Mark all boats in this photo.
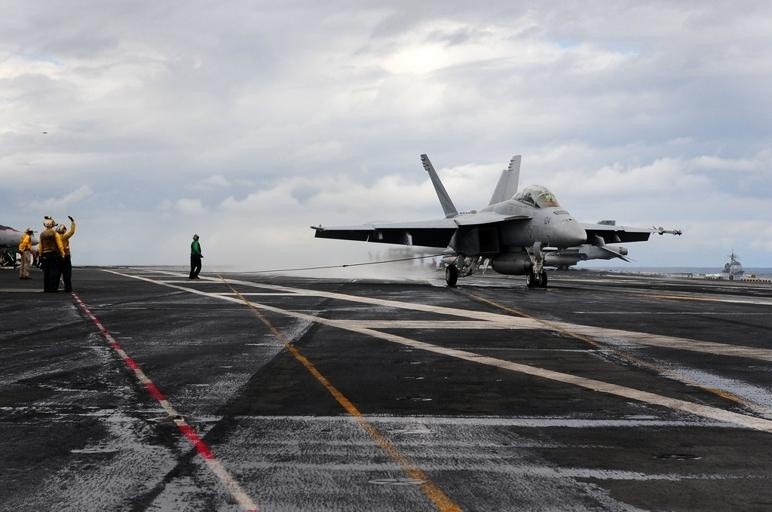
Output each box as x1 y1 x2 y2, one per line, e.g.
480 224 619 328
725 251 744 274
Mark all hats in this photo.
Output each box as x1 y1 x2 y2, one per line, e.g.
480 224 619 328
25 227 34 233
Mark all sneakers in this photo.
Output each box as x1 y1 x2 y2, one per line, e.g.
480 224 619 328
190 272 200 280
18 274 32 279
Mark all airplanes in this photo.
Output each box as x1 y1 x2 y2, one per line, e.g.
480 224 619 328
0 225 42 265
310 154 683 286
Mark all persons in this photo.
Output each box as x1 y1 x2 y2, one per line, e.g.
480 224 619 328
188 234 203 280
18 215 76 294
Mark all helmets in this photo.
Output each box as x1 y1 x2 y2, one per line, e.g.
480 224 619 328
43 218 67 233
192 234 200 240
